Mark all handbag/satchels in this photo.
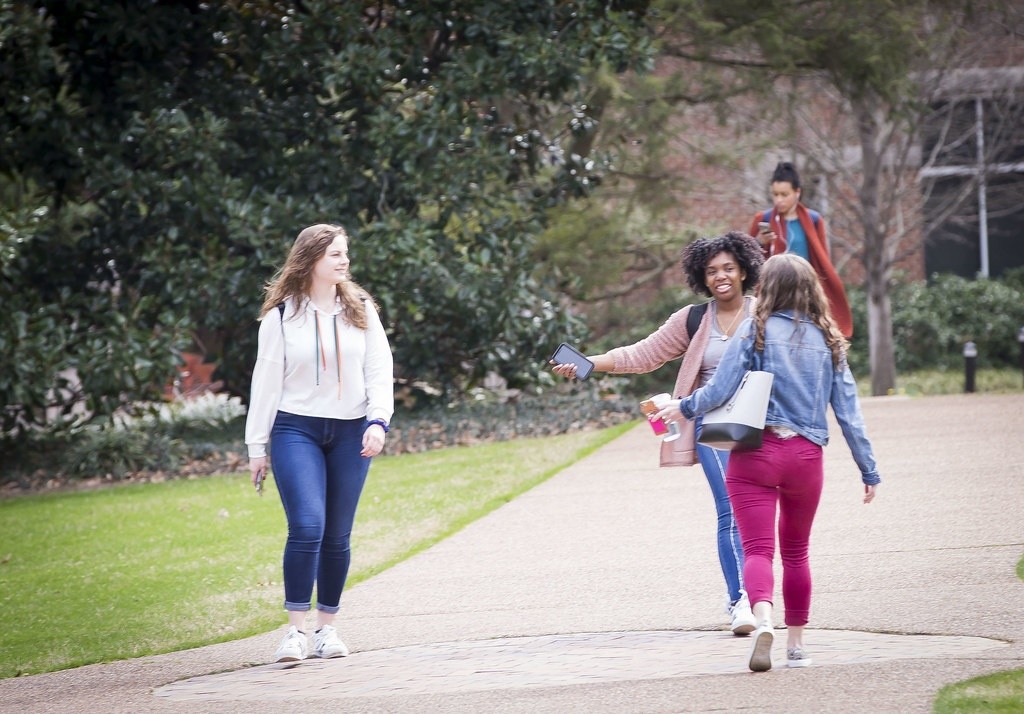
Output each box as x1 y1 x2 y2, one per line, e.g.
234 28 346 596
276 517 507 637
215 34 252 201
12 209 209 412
697 369 775 453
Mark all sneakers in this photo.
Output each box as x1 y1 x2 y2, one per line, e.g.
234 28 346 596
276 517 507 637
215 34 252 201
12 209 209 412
745 620 774 673
272 625 309 663
727 590 757 636
306 624 349 660
784 645 810 668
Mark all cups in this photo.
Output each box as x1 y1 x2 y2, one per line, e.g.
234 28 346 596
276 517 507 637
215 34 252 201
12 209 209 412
647 392 681 443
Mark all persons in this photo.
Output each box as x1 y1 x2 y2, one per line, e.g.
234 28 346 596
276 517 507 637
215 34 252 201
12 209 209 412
651 254 881 672
549 232 761 636
242 224 394 664
747 162 855 341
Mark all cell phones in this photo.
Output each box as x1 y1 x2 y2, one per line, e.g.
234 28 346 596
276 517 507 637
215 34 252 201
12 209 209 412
640 400 668 438
757 222 770 233
257 472 263 497
551 342 594 382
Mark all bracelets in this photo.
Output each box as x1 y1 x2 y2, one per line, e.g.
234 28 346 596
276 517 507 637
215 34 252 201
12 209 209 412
366 420 390 433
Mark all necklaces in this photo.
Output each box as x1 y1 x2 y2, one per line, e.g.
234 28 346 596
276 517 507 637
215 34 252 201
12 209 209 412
715 295 746 342
